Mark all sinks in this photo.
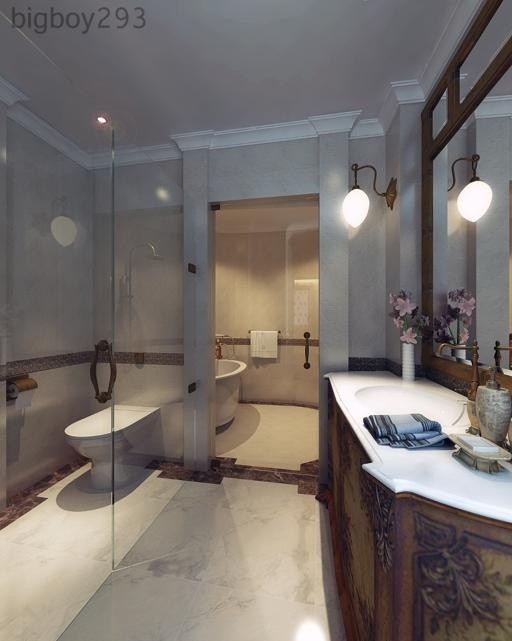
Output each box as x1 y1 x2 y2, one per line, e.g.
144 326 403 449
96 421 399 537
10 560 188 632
354 384 468 438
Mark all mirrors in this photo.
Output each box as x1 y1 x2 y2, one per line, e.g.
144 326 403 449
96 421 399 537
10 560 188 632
420 1 512 376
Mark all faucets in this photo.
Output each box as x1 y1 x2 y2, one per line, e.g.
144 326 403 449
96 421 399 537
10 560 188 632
492 340 511 373
437 338 480 402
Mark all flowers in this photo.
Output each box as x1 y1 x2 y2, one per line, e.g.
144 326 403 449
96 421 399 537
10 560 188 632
433 286 479 348
388 288 431 344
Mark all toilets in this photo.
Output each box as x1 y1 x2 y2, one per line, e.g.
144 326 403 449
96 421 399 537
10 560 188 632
63 403 161 491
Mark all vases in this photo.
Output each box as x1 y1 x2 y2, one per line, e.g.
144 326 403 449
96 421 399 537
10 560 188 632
449 340 469 359
399 335 415 380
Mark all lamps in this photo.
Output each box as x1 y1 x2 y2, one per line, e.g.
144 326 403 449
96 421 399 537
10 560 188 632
342 163 396 228
447 153 497 221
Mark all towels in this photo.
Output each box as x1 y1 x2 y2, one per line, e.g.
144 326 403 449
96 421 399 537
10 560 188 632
249 330 278 358
363 413 457 450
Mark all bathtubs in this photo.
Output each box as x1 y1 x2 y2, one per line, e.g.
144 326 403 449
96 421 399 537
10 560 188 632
215 357 248 434
